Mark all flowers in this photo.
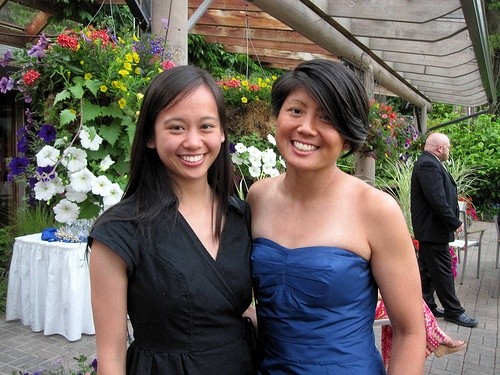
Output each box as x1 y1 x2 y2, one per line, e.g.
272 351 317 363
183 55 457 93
0 18 419 227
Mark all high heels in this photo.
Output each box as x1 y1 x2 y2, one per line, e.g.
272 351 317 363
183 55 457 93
434 339 467 358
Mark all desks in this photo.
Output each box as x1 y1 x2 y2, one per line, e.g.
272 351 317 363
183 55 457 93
6 232 136 341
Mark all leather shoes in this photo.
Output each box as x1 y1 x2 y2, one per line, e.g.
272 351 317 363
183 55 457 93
433 304 479 327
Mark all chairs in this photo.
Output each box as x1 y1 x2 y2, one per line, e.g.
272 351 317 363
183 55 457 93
449 210 487 285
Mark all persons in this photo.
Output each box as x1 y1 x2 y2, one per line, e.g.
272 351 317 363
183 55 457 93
410 132 479 328
371 285 467 375
87 66 269 375
245 59 427 375
